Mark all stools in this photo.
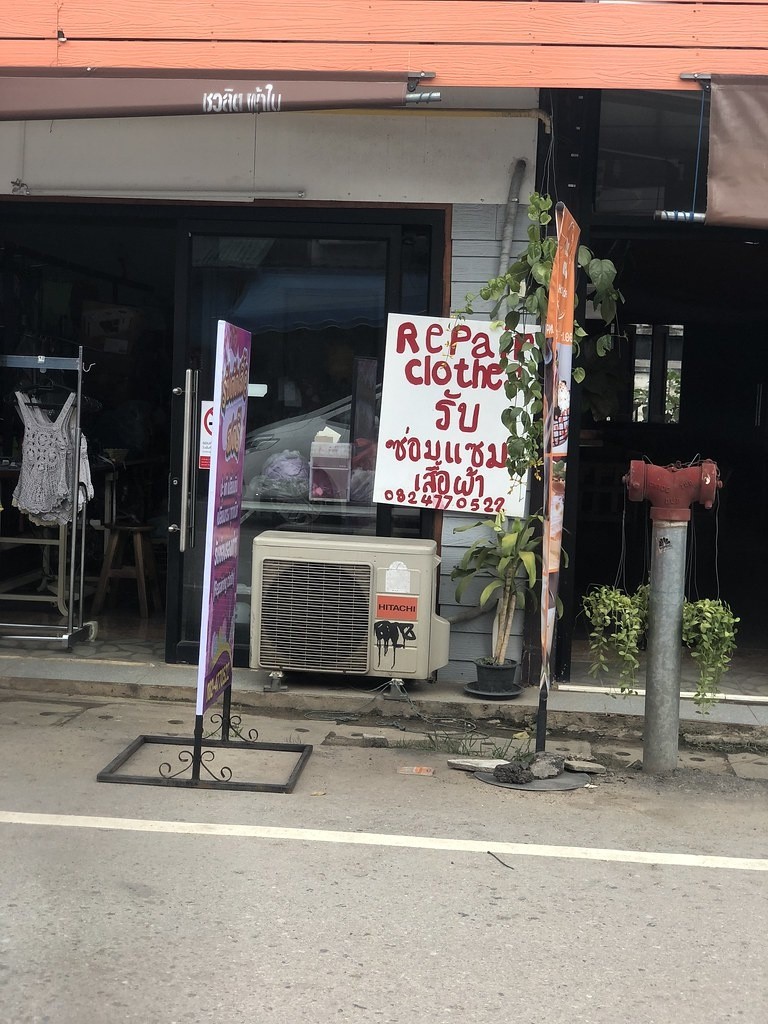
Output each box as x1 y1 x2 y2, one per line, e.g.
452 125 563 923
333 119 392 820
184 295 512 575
90 521 164 623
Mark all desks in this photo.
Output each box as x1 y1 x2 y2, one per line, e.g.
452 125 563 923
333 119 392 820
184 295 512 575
0 452 160 600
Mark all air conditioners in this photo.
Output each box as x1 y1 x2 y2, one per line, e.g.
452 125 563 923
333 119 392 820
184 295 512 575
247 528 452 681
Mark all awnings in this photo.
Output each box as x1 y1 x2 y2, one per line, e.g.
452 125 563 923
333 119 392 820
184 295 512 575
653 71 767 228
1 64 441 120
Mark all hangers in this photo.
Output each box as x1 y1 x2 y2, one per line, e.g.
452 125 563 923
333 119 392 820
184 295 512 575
4 364 104 411
